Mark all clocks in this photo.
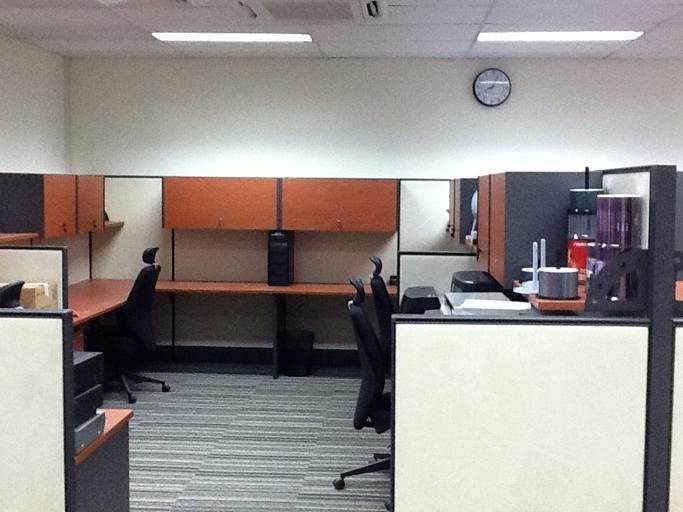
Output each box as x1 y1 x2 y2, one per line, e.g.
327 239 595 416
473 68 512 107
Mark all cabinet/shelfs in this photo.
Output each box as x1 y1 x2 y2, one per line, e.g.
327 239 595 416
161 175 279 233
477 171 603 290
0 172 105 239
278 176 400 234
447 179 478 244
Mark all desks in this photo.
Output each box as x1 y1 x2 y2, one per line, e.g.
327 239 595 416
74 408 134 512
68 278 397 379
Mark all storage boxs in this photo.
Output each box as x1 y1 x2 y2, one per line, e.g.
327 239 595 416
19 280 58 311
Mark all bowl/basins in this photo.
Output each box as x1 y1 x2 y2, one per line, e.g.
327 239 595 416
537 267 578 298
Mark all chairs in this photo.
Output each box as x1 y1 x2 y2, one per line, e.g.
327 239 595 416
369 255 398 379
89 246 171 403
333 277 393 510
